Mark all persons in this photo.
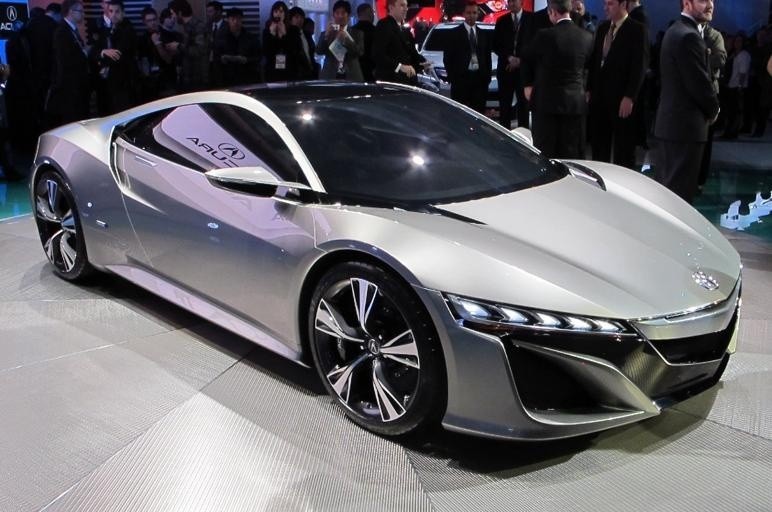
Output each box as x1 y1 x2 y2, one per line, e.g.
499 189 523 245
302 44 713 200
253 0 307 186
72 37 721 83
0 1 771 206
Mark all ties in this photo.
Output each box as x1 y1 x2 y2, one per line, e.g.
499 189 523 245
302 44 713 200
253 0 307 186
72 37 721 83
514 14 518 31
470 28 476 54
601 24 616 67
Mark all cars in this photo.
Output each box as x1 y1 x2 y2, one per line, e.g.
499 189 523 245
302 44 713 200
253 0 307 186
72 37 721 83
30 79 744 450
417 19 517 120
